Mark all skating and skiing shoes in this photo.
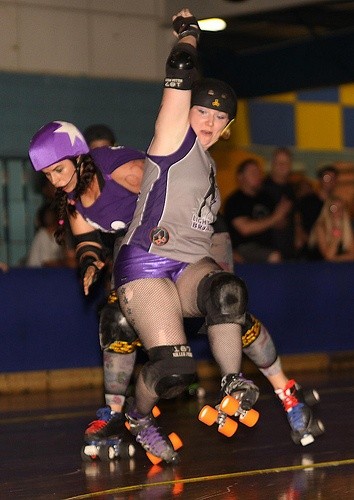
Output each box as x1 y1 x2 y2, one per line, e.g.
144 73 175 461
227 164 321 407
83 407 138 460
274 379 324 446
199 373 260 437
125 406 183 465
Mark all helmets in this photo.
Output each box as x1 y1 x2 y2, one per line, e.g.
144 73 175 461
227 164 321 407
191 78 237 120
29 121 90 172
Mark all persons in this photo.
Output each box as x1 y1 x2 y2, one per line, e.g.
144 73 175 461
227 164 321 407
84 125 116 150
27 205 65 269
26 120 320 461
112 10 260 468
217 146 354 263
